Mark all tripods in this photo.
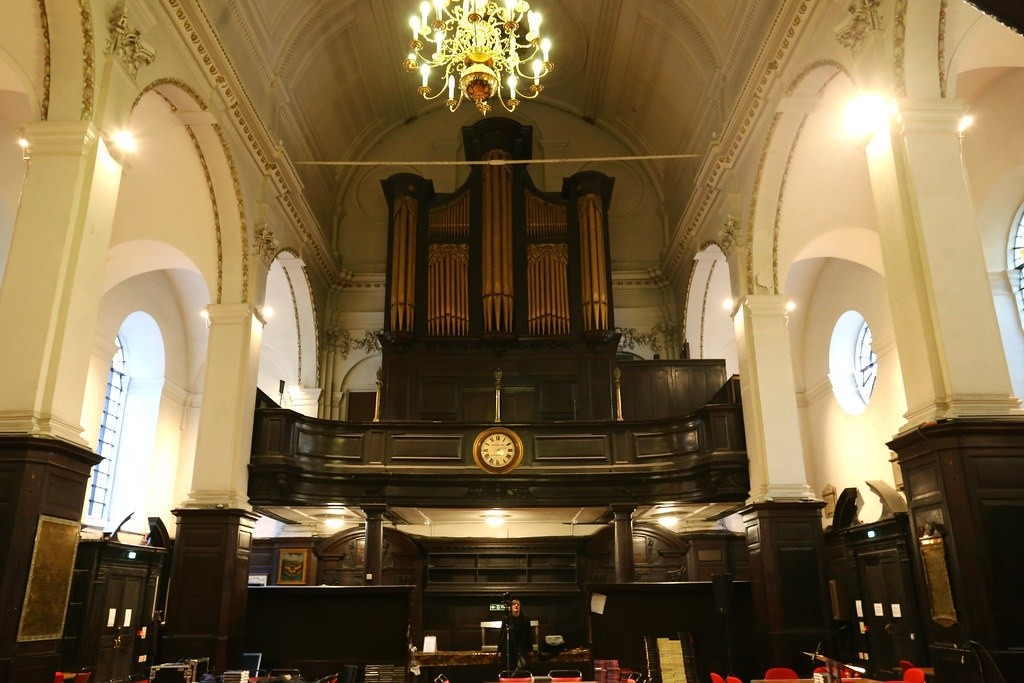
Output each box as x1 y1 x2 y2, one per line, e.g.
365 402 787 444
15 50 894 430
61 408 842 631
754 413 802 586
484 618 525 683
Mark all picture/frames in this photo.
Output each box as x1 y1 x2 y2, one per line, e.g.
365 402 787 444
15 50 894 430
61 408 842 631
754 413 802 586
275 549 308 584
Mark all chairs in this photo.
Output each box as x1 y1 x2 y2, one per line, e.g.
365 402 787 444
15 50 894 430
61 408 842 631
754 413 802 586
55 667 339 683
433 670 643 683
710 661 925 683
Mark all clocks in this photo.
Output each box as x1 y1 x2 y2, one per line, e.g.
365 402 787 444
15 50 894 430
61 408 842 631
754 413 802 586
473 427 524 474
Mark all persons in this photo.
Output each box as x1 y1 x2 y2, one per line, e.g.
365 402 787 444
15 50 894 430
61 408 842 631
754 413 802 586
498 598 536 674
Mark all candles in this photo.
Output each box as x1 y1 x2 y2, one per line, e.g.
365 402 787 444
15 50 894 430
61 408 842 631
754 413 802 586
409 0 553 102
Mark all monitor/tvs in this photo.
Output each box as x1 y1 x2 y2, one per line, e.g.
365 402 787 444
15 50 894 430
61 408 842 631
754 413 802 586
101 512 134 534
866 480 907 514
148 517 170 549
832 487 865 527
